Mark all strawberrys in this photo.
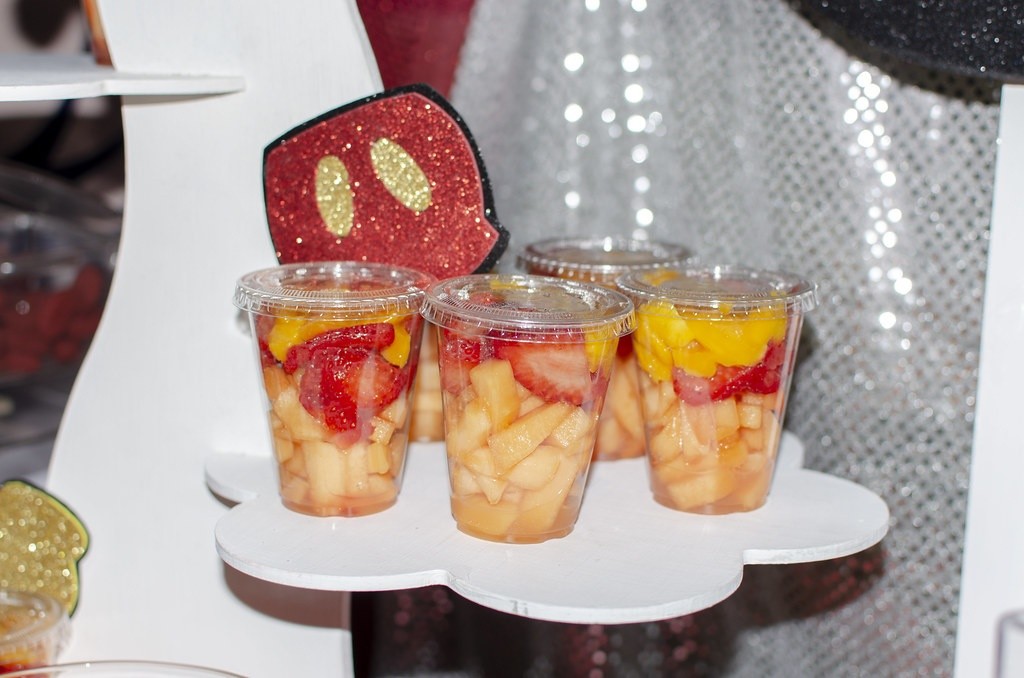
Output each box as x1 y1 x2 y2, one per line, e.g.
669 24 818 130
255 281 792 433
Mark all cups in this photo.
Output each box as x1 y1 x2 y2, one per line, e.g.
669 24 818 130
0 589 65 678
234 235 819 545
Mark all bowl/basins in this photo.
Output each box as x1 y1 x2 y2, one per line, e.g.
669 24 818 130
0 661 246 678
